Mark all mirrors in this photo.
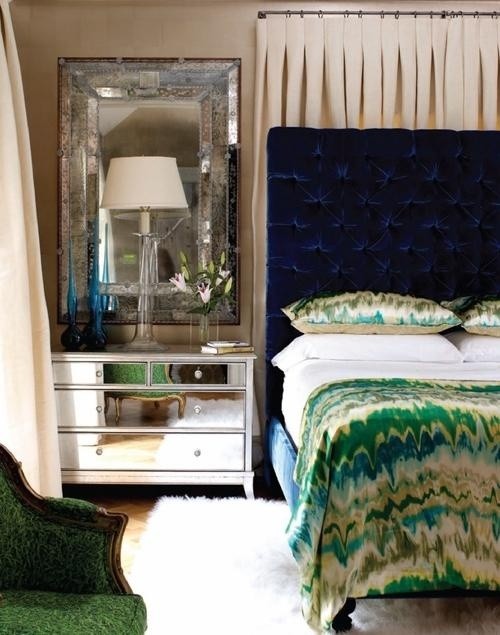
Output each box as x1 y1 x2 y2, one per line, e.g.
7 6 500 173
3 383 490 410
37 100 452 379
56 58 239 326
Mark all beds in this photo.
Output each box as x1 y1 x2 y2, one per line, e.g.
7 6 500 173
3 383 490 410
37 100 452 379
267 363 498 632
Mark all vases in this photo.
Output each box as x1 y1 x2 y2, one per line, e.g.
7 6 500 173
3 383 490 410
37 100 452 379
199 311 218 344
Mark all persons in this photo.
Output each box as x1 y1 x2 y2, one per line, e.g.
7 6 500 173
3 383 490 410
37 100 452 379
156 247 179 283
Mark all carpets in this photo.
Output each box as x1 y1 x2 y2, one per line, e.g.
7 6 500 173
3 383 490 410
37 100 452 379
141 489 498 632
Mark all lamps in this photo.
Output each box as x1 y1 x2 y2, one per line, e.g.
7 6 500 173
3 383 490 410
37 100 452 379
99 156 190 353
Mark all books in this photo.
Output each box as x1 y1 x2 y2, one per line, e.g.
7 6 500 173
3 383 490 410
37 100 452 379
208 340 250 348
199 344 254 355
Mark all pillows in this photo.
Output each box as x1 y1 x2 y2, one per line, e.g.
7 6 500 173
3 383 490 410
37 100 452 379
274 291 499 367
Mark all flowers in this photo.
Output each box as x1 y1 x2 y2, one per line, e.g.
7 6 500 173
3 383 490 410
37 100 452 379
174 249 238 314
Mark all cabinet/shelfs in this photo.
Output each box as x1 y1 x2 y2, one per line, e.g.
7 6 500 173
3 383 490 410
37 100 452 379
53 345 258 500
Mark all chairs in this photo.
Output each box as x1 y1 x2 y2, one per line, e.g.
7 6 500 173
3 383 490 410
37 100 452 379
0 446 146 632
